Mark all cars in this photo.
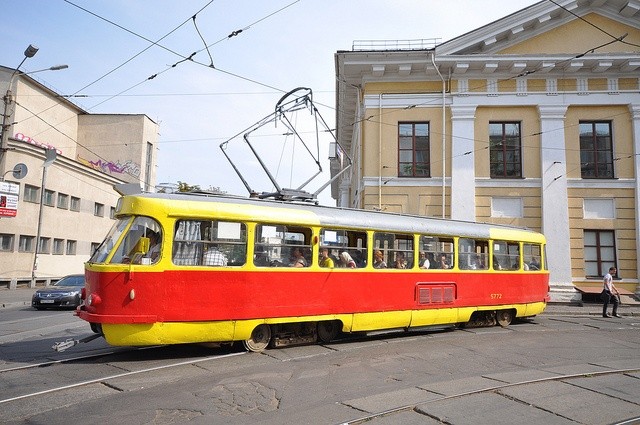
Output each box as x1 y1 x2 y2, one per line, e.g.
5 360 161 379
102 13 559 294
31 274 85 310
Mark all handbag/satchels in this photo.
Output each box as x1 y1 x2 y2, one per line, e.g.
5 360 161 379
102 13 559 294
600 284 611 303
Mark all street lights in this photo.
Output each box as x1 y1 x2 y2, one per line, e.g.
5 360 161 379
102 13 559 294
1 64 69 157
0 43 39 156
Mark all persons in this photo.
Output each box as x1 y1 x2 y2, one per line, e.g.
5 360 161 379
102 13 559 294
427 252 435 269
475 256 485 269
602 267 622 318
335 247 365 268
435 253 449 268
529 256 540 270
373 250 387 268
203 243 228 266
515 257 529 270
140 230 162 264
392 251 409 269
292 246 308 267
254 246 266 266
418 251 431 269
318 249 335 268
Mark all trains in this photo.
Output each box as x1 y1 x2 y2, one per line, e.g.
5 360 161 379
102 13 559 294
75 183 550 354
